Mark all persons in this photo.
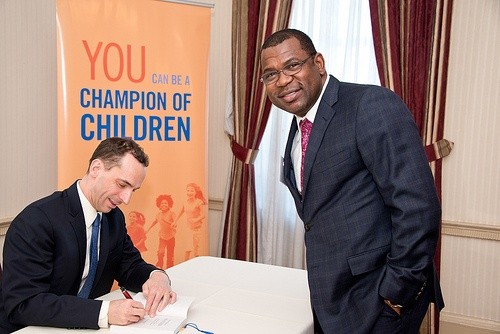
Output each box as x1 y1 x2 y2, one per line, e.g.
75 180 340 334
127 183 207 268
0 137 176 334
260 29 442 334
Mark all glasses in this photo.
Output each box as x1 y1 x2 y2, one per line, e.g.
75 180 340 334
260 53 316 86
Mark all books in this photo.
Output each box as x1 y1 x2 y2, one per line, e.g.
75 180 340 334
109 290 195 334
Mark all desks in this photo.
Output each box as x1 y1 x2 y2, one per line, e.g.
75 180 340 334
8 256 314 334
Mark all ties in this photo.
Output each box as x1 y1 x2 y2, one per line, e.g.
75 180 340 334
77 213 100 299
299 118 313 200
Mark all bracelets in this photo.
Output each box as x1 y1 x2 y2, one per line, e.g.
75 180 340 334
385 300 402 307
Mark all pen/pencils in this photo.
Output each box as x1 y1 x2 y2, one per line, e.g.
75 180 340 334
120 286 132 299
